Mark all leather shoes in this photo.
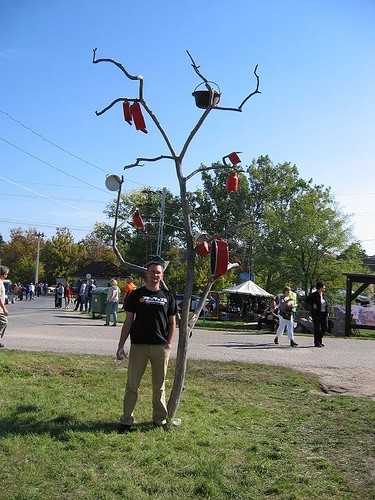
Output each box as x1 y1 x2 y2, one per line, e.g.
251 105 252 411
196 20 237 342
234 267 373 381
315 341 324 346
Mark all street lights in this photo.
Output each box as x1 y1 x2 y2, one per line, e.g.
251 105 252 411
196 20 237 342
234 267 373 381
35 231 45 284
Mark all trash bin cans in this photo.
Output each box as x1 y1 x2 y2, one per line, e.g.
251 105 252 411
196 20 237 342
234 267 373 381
90 287 120 319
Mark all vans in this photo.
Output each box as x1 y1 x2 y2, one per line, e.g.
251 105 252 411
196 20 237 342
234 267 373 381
3 280 16 304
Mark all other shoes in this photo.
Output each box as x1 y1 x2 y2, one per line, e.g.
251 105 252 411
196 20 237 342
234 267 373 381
274 337 279 344
104 323 109 325
290 339 299 347
118 424 133 433
112 323 117 326
157 424 168 430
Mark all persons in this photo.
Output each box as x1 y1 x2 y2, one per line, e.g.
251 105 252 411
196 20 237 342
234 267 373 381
104 279 120 326
35 281 48 297
0 265 10 347
304 281 329 347
116 254 178 433
274 286 298 347
54 282 73 309
121 276 136 303
258 283 297 332
26 283 35 301
212 303 240 316
9 282 24 304
3 282 8 305
74 279 96 313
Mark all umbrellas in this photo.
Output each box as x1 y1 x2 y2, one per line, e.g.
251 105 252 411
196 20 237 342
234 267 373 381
220 280 275 310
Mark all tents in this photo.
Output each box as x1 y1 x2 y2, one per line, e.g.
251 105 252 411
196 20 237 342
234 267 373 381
340 290 371 305
